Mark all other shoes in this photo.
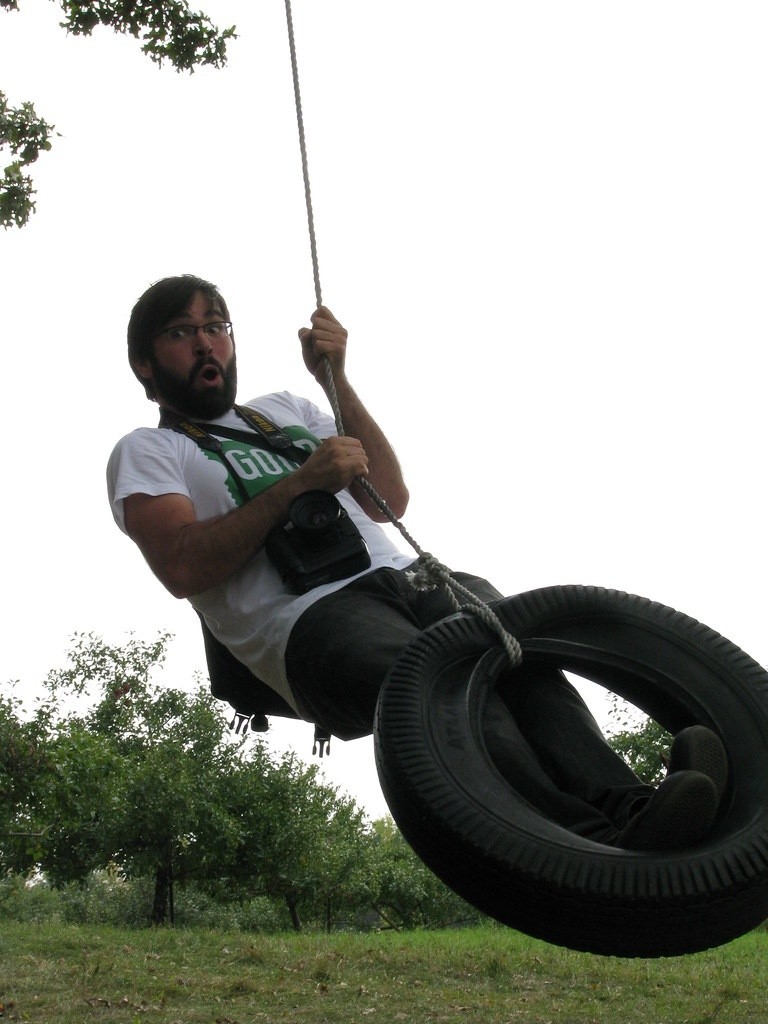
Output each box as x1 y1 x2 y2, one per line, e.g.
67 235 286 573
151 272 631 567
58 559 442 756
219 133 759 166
615 770 719 852
665 725 728 798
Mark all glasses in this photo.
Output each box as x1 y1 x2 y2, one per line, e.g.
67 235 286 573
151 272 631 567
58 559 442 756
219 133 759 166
149 320 232 343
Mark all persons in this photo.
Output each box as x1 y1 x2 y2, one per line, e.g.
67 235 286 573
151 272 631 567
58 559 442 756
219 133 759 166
107 274 728 852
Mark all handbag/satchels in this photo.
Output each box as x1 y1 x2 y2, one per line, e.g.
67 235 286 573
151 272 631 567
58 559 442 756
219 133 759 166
192 606 331 759
